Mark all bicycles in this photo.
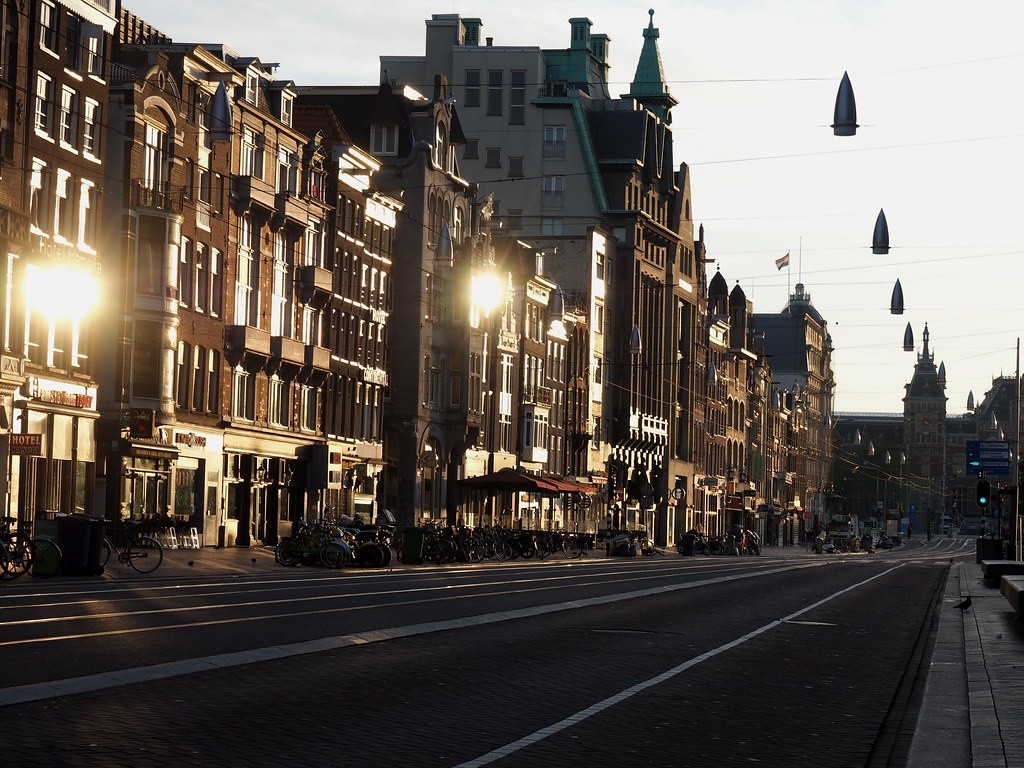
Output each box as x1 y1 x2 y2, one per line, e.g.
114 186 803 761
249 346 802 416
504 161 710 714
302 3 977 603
277 505 397 569
78 512 164 574
402 520 587 564
673 527 729 555
0 514 64 579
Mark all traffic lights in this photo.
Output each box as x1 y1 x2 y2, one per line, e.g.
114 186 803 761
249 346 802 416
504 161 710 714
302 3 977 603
976 480 991 509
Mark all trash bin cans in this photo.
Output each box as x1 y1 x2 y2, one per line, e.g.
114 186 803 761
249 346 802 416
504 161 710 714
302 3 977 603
35 510 106 577
975 536 1003 564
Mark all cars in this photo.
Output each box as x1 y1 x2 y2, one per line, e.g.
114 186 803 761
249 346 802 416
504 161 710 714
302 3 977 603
983 533 1000 540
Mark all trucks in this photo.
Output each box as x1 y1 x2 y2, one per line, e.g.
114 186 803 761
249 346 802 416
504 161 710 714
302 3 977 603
938 516 961 534
828 514 869 540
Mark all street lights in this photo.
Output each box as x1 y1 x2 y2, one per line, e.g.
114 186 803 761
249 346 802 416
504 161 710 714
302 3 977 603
997 488 1008 540
550 320 573 530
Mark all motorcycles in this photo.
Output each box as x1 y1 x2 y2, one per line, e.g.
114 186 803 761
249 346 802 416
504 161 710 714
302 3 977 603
876 535 894 548
